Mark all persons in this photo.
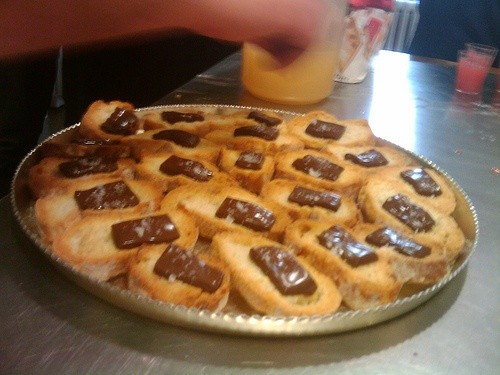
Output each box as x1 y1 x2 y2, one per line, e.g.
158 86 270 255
1 1 342 215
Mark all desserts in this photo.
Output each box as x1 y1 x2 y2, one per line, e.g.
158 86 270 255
31 98 467 320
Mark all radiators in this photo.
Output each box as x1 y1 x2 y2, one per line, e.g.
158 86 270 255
380 1 420 53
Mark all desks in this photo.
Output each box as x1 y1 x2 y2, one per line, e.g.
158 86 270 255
1 44 499 375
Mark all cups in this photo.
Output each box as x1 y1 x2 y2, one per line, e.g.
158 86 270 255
240 0 347 106
456 49 492 95
465 42 498 70
